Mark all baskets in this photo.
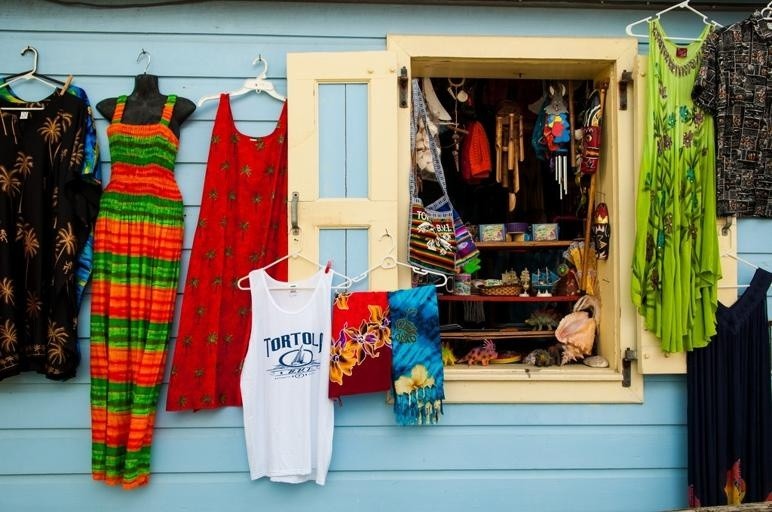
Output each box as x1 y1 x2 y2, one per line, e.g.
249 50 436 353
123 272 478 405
477 283 523 296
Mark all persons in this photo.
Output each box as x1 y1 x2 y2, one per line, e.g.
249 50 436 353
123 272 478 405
95 74 197 488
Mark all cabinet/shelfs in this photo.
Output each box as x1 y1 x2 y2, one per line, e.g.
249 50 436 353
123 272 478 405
412 225 612 372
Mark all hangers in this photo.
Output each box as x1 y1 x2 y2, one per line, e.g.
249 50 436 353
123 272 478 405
759 1 772 23
0 45 80 115
712 228 772 289
334 231 450 292
192 51 289 119
238 228 353 293
627 1 727 42
